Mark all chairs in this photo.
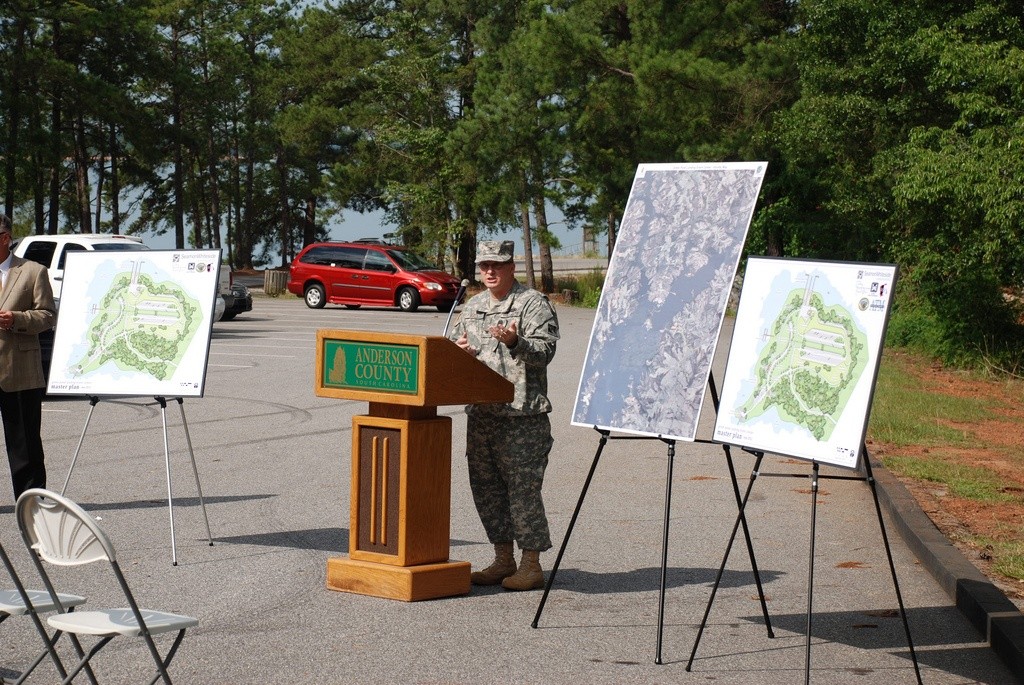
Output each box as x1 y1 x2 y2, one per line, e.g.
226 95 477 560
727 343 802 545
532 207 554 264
0 541 87 685
15 488 199 685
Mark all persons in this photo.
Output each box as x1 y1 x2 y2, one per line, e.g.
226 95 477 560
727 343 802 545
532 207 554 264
0 215 58 505
449 239 562 588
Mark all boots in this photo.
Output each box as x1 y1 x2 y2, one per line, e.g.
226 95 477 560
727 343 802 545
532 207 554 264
470 540 544 589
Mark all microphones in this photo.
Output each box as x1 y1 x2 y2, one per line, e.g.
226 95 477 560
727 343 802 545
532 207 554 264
455 279 470 302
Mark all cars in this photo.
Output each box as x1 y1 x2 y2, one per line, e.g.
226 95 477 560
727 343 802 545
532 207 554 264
220 281 253 320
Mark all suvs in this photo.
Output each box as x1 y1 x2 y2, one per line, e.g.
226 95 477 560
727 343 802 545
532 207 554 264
14 233 225 324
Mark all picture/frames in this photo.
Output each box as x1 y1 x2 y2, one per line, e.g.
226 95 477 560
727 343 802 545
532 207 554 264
46 248 223 399
712 255 899 471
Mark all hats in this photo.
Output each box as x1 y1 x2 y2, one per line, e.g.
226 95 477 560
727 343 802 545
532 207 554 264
474 239 515 263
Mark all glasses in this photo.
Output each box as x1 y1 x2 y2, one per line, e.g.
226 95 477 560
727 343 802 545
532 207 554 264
478 262 503 269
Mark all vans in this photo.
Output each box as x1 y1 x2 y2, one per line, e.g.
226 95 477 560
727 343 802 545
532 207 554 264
288 240 467 313
